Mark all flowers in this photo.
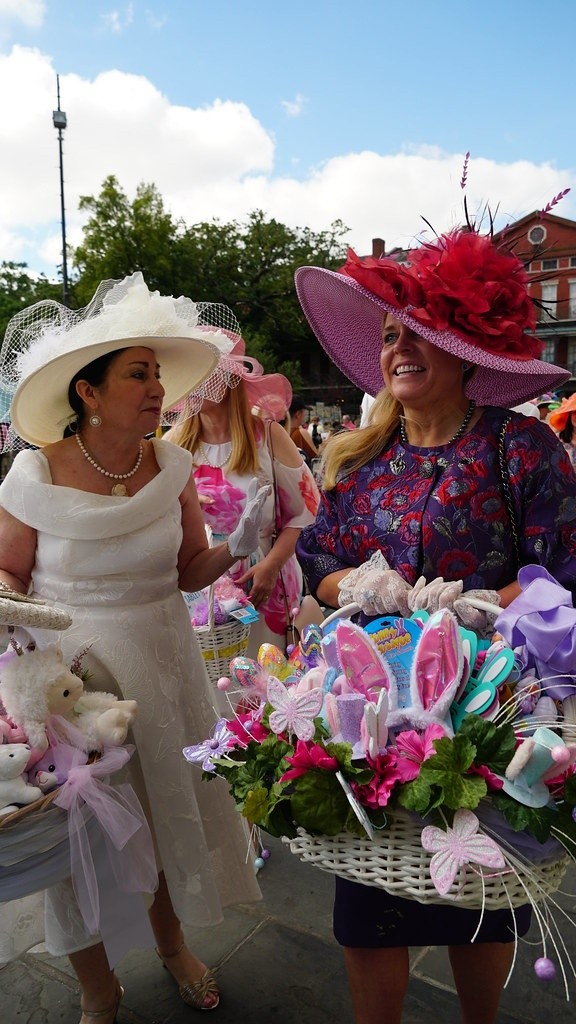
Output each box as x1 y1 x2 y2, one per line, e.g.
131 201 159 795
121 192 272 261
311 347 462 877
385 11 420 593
343 226 555 356
185 668 575 837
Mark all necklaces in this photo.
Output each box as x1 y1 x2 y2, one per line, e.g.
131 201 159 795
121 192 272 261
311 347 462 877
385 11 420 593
199 439 234 470
400 396 476 449
75 430 144 479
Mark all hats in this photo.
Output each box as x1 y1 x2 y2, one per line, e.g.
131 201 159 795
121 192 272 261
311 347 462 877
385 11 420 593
160 327 291 426
294 266 571 412
551 395 576 430
10 313 221 449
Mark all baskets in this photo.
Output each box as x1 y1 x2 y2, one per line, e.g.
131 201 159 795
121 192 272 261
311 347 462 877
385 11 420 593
0 638 106 903
188 579 246 688
287 597 569 908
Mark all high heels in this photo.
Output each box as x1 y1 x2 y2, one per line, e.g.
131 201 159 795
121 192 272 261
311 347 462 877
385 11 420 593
78 972 124 1024
156 938 219 1011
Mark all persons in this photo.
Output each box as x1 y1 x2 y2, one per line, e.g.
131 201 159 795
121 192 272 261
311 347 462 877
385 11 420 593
0 343 274 1024
157 300 324 861
0 392 576 600
293 315 576 1024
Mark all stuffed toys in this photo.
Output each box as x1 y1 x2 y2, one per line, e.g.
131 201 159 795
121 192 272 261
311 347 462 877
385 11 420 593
0 643 137 816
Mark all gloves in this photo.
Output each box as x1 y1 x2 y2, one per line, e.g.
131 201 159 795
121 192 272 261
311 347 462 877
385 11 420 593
227 477 270 558
